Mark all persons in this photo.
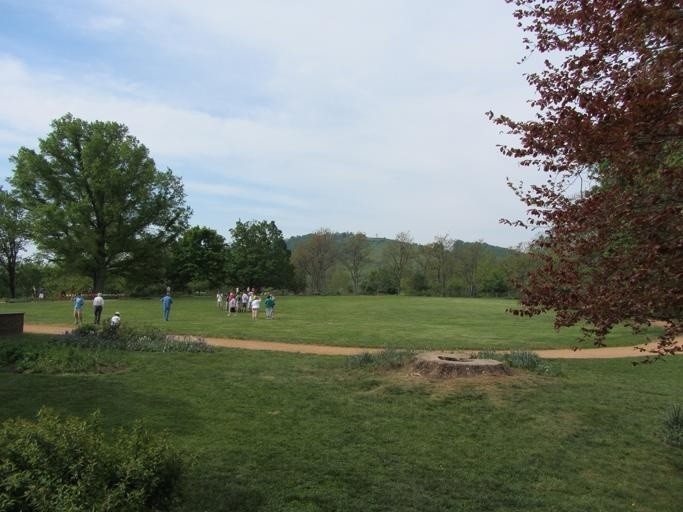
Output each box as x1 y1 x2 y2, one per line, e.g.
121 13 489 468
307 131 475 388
111 312 121 327
216 287 275 320
72 293 84 324
93 293 104 325
160 292 173 321
